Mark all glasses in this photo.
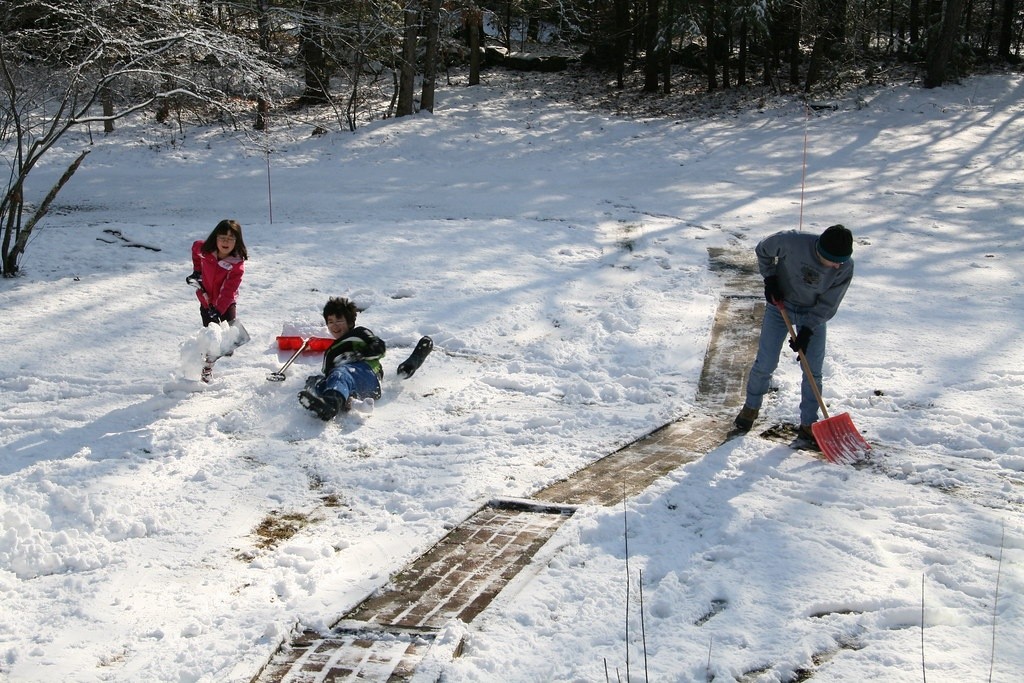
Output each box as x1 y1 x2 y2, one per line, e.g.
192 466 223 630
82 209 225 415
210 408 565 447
217 236 236 244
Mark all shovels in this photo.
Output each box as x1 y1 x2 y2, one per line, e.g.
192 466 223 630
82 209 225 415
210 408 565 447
185 271 253 367
764 293 874 467
267 330 338 387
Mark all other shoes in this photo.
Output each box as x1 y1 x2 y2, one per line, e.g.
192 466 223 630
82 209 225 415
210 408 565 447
798 423 817 445
201 367 212 383
298 390 336 422
734 404 759 432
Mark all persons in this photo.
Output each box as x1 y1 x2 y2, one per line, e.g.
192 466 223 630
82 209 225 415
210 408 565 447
186 220 248 382
298 296 386 421
734 224 854 439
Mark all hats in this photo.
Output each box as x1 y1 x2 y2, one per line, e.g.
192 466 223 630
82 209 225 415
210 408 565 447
817 224 853 263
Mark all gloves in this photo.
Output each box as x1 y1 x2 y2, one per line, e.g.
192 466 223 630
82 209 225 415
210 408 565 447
788 324 813 362
207 308 221 322
763 276 782 304
186 272 198 284
